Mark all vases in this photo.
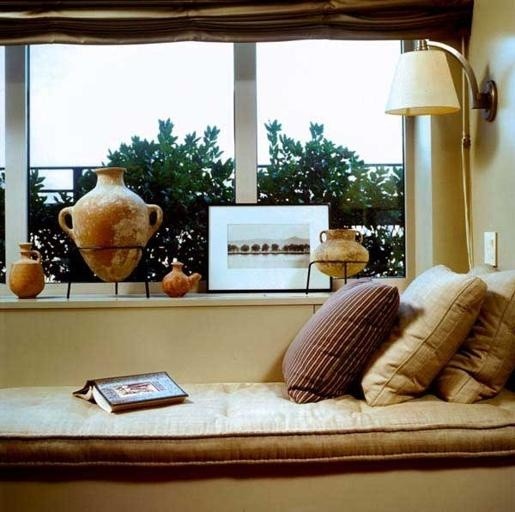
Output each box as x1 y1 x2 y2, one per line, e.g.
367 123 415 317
161 261 201 298
313 227 370 278
59 165 166 285
8 243 46 300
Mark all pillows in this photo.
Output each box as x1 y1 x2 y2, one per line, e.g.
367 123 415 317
367 263 479 407
446 271 515 404
277 277 399 404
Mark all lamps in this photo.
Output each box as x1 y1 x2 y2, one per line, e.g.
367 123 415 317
385 36 499 127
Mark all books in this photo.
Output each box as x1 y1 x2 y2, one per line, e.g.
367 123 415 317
72 367 190 415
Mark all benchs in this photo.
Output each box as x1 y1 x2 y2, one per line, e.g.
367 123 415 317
0 381 515 511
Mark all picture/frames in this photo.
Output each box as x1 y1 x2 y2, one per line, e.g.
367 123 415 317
206 201 332 290
